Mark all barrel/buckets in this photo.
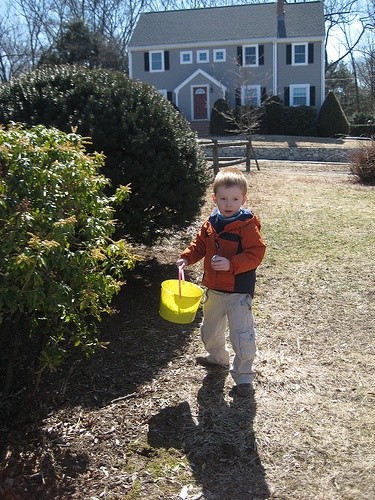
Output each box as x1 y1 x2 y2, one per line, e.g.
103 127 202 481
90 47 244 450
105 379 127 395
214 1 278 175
160 265 204 323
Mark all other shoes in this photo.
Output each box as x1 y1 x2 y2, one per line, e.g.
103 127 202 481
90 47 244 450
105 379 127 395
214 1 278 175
236 383 254 398
194 354 221 366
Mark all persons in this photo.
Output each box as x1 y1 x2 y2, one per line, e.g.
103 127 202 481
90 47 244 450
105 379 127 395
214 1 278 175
176 166 266 396
